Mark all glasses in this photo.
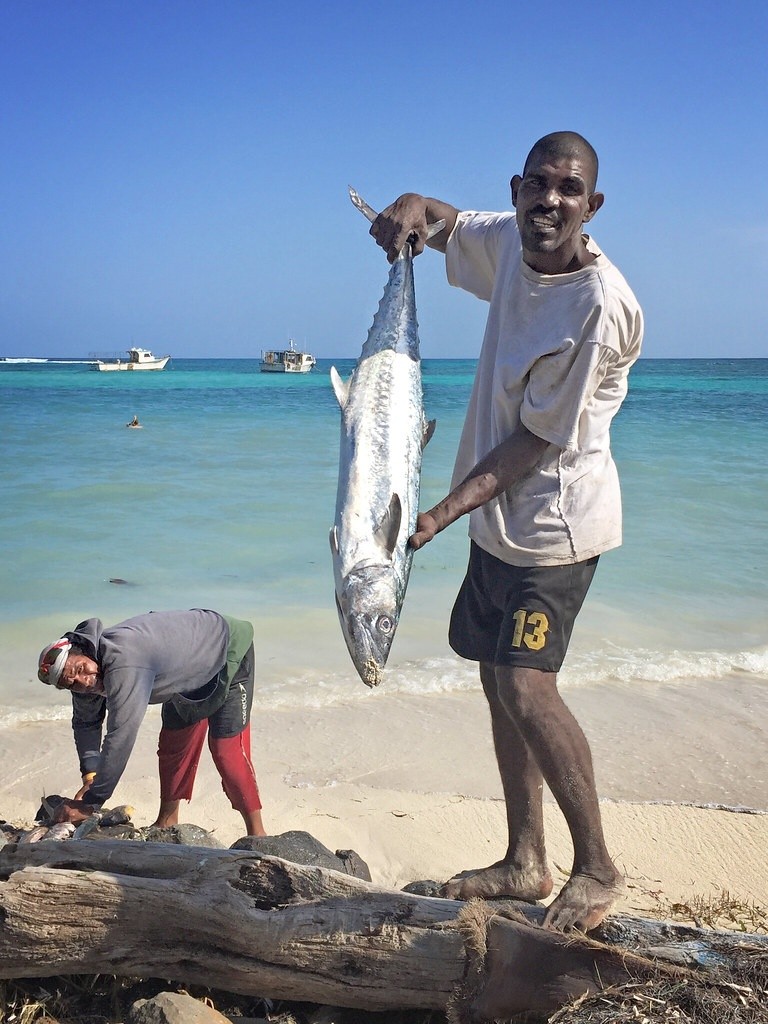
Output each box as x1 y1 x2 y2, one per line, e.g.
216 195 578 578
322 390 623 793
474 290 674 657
38 641 69 685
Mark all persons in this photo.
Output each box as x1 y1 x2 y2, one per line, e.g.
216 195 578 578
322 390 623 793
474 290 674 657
38 608 268 835
368 132 646 933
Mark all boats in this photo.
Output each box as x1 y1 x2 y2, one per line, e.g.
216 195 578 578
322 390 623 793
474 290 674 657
258 340 317 374
87 348 171 371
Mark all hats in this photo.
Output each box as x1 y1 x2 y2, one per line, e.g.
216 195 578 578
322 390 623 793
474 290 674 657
38 638 73 690
